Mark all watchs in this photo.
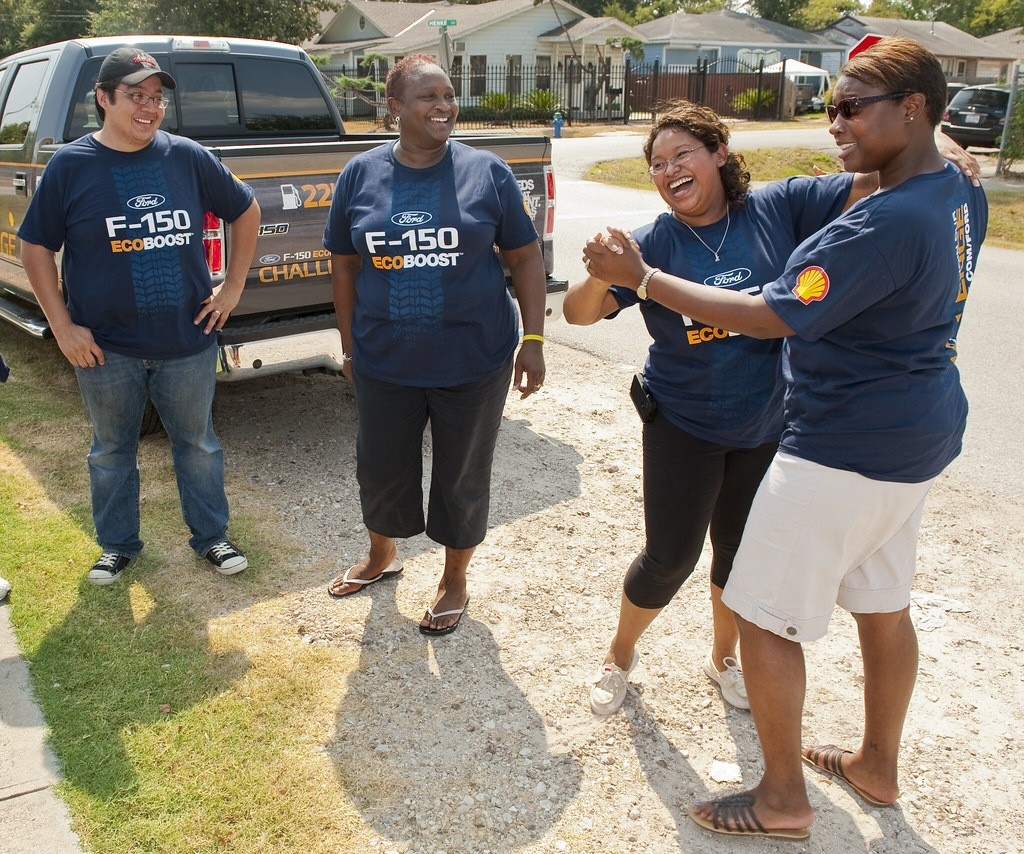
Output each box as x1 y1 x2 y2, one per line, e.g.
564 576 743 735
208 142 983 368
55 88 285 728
637 268 660 301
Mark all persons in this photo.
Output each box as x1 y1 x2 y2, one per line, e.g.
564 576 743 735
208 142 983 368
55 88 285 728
17 48 261 585
563 101 983 716
322 52 546 635
583 39 988 841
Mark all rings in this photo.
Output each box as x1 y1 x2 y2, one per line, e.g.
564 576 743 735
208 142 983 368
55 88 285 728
215 311 220 315
535 383 541 388
584 259 591 269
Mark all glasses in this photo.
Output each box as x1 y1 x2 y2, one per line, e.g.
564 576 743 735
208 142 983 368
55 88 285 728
827 91 932 124
112 88 170 109
648 144 704 176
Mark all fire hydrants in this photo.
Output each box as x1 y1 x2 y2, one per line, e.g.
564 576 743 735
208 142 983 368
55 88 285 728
552 112 564 138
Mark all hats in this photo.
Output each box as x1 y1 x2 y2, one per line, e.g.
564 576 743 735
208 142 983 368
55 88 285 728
97 47 178 90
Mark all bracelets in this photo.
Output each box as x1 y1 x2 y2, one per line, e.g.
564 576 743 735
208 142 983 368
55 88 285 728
342 353 352 362
522 335 545 344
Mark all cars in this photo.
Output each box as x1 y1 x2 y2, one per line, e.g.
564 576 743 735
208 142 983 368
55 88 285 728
941 83 1024 150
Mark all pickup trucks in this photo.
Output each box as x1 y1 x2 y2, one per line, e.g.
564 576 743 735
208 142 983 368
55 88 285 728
0 36 569 438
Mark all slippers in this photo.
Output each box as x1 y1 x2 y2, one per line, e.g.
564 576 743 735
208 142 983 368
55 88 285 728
419 591 471 636
801 743 892 808
687 789 811 840
328 557 405 598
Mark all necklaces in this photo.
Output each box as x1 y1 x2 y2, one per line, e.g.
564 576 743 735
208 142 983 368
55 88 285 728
682 200 730 262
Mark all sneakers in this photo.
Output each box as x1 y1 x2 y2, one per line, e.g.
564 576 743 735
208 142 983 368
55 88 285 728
703 647 750 710
590 646 640 714
205 539 249 575
87 552 130 586
